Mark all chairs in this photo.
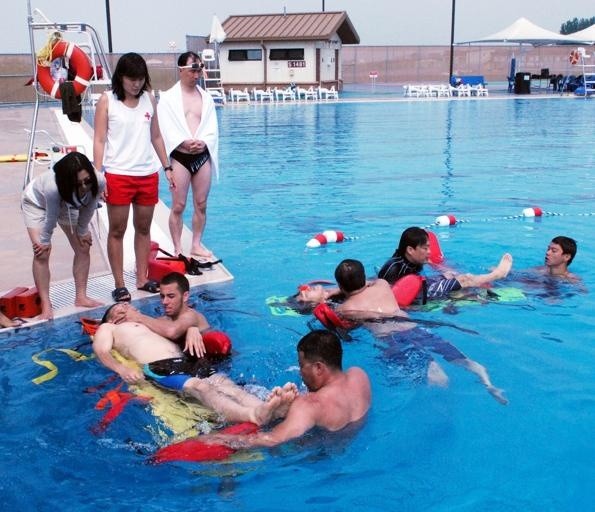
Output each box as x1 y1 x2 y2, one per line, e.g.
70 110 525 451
577 47 591 59
88 85 340 104
506 73 595 97
402 76 490 97
25 0 116 93
202 48 215 62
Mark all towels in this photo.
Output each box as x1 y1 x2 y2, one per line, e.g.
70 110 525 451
155 78 221 179
58 82 83 124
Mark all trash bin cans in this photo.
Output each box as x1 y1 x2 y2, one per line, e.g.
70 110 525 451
514 72 531 94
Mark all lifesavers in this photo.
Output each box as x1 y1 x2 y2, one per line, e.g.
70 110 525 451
36 42 92 99
569 51 578 64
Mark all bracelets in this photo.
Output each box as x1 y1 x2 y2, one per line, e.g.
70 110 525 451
163 163 175 172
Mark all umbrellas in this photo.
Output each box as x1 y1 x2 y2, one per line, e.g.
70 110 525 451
207 11 228 78
454 14 595 82
564 20 594 44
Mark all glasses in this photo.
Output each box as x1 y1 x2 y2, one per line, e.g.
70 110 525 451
178 62 208 71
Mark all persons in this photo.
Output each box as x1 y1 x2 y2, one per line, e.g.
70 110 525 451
518 234 589 305
0 310 24 332
374 224 435 287
285 252 515 315
110 270 234 370
20 151 106 321
157 45 220 262
92 52 178 303
325 259 511 407
88 301 300 429
191 328 374 460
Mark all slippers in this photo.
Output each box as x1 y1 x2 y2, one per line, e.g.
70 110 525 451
136 279 162 294
110 287 131 302
